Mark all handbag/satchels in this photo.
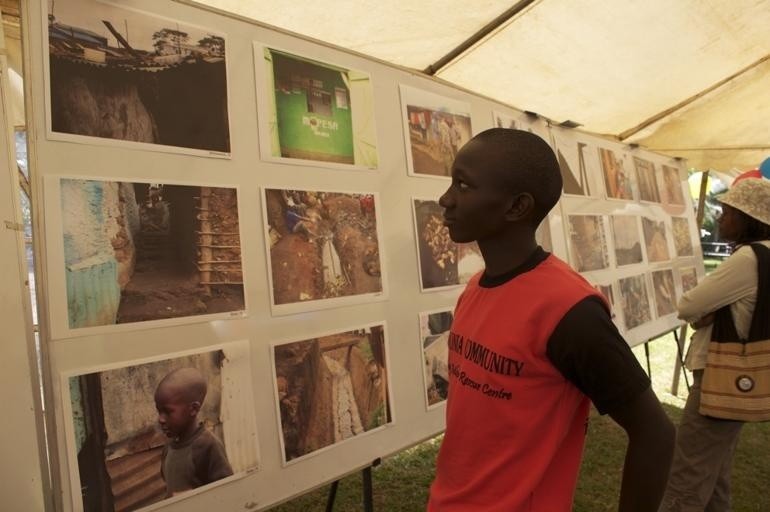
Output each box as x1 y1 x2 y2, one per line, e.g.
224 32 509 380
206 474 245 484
698 337 770 422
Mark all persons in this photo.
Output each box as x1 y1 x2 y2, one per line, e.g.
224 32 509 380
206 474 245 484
427 128 676 512
655 177 770 511
154 367 235 499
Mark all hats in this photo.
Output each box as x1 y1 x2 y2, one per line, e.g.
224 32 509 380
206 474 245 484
714 177 769 225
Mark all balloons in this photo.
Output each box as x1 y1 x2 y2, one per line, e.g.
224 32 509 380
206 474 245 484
732 168 760 187
759 156 770 179
